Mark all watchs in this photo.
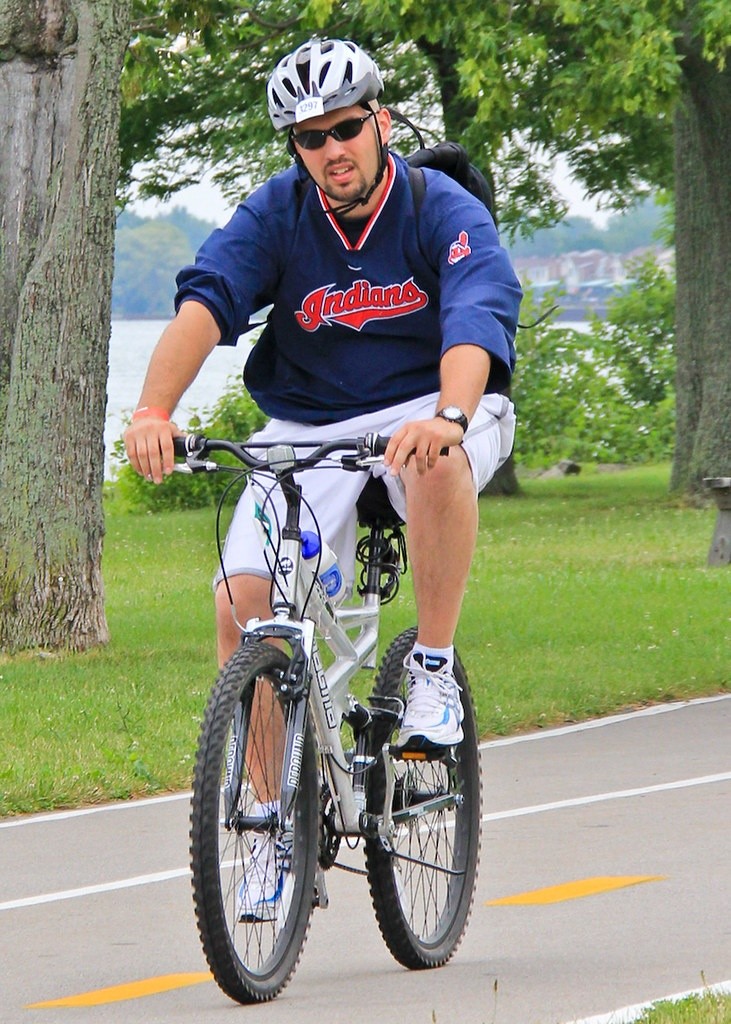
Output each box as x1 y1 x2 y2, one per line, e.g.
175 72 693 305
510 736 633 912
436 406 469 435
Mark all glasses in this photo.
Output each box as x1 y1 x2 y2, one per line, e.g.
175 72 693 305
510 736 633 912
289 111 377 151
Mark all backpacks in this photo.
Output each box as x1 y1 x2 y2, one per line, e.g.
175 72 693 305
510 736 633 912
291 141 495 267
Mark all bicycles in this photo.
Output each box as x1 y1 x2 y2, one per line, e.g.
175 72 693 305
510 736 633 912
156 430 484 1007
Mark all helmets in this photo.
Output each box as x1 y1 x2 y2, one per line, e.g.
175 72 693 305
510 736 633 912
265 38 384 132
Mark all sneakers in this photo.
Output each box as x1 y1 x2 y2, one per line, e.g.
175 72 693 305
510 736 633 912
399 672 467 748
236 859 284 920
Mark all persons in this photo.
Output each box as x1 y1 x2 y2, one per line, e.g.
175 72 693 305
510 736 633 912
121 37 521 924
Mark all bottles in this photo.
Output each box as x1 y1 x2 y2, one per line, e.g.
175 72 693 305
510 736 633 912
300 530 347 604
